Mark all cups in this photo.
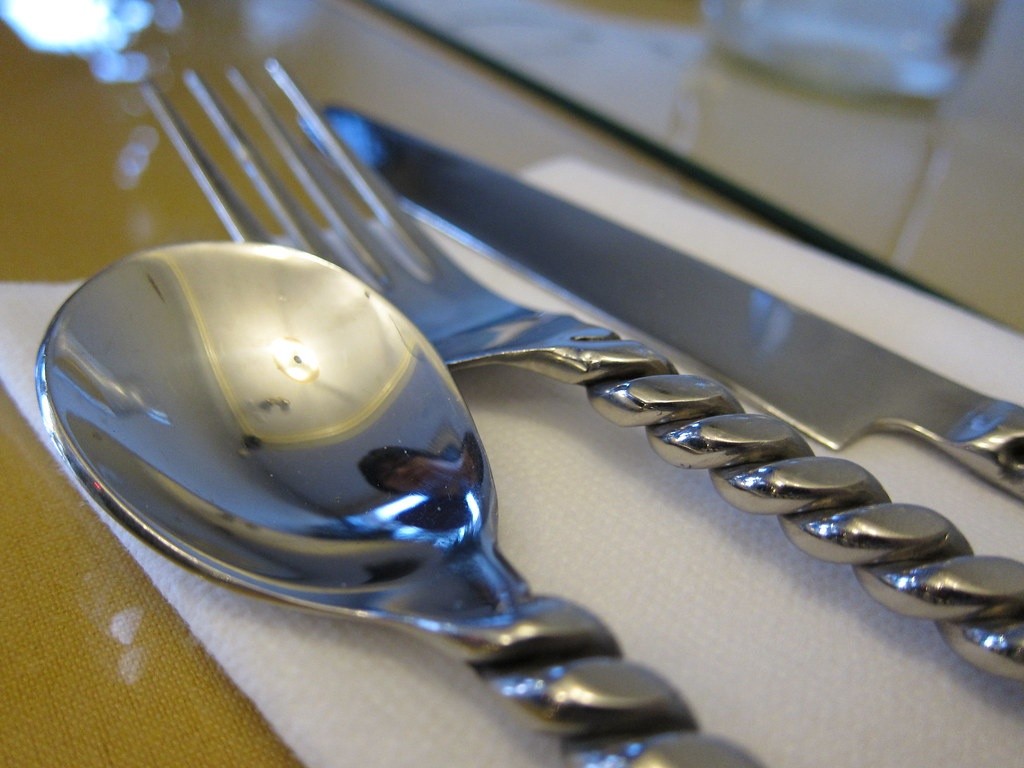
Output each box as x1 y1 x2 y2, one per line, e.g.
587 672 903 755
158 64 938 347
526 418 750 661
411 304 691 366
702 0 1002 115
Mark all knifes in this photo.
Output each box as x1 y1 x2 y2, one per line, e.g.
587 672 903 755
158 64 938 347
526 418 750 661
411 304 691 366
293 100 1024 504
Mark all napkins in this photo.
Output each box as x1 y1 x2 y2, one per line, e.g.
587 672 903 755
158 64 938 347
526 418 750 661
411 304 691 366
1 159 1024 768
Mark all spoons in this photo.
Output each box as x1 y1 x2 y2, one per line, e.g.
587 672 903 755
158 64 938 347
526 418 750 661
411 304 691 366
33 240 766 768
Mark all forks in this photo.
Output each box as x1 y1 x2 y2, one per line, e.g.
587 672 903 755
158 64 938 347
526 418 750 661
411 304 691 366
140 54 1024 683
